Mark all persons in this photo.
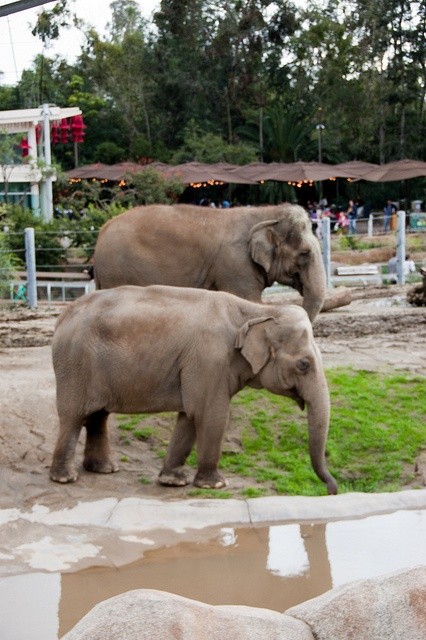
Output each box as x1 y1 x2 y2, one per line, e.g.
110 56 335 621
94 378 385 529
203 197 400 232
388 253 399 274
403 255 416 276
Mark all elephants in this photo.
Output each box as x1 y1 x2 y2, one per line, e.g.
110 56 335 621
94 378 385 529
47 284 338 496
89 201 327 325
